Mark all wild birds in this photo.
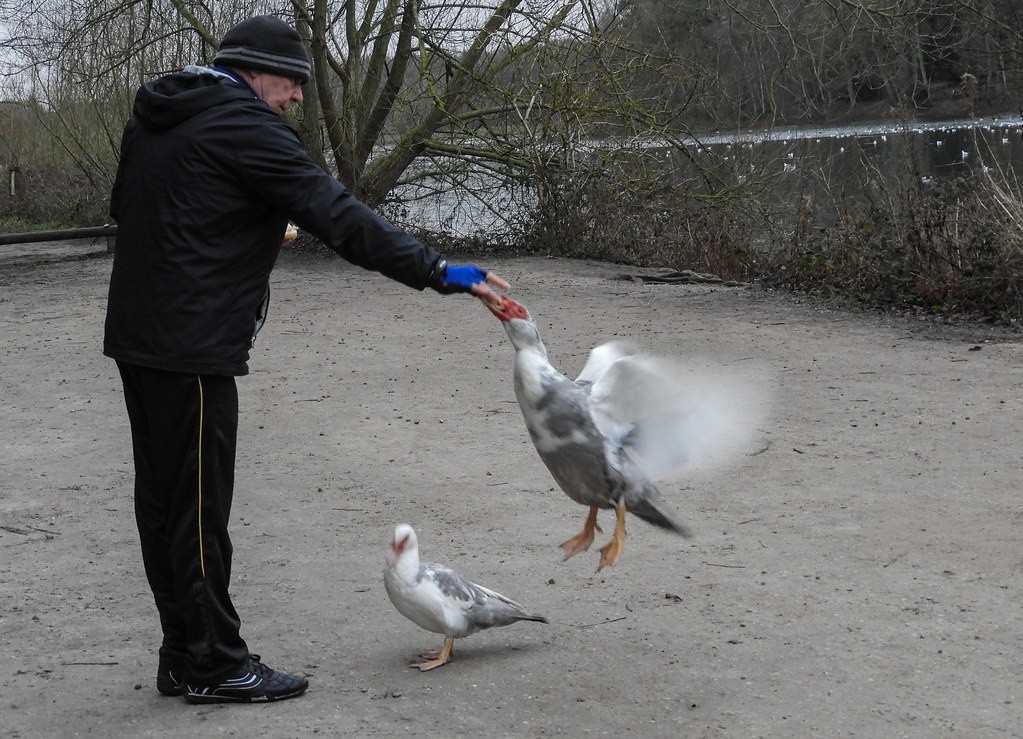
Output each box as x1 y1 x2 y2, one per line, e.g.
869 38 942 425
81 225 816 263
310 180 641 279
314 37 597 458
382 522 551 673
486 294 762 575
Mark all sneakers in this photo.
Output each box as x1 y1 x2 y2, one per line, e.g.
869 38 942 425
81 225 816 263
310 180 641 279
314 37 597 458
154 647 310 705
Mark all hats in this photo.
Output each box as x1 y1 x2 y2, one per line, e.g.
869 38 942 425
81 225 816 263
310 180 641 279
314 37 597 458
208 13 313 84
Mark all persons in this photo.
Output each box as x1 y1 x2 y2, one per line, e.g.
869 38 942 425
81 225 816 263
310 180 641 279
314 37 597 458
100 12 514 702
